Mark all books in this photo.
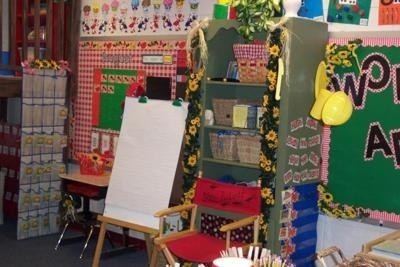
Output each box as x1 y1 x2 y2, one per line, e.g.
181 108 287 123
371 238 400 261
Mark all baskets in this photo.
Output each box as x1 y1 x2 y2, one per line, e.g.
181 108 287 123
236 135 260 164
234 43 267 83
208 132 238 161
211 97 238 126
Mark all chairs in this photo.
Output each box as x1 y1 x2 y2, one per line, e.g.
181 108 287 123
145 172 265 267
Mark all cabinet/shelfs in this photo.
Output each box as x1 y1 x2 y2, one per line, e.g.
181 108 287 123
1 62 68 243
198 17 330 265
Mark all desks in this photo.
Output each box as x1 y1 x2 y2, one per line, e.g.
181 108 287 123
314 227 399 267
51 167 116 260
